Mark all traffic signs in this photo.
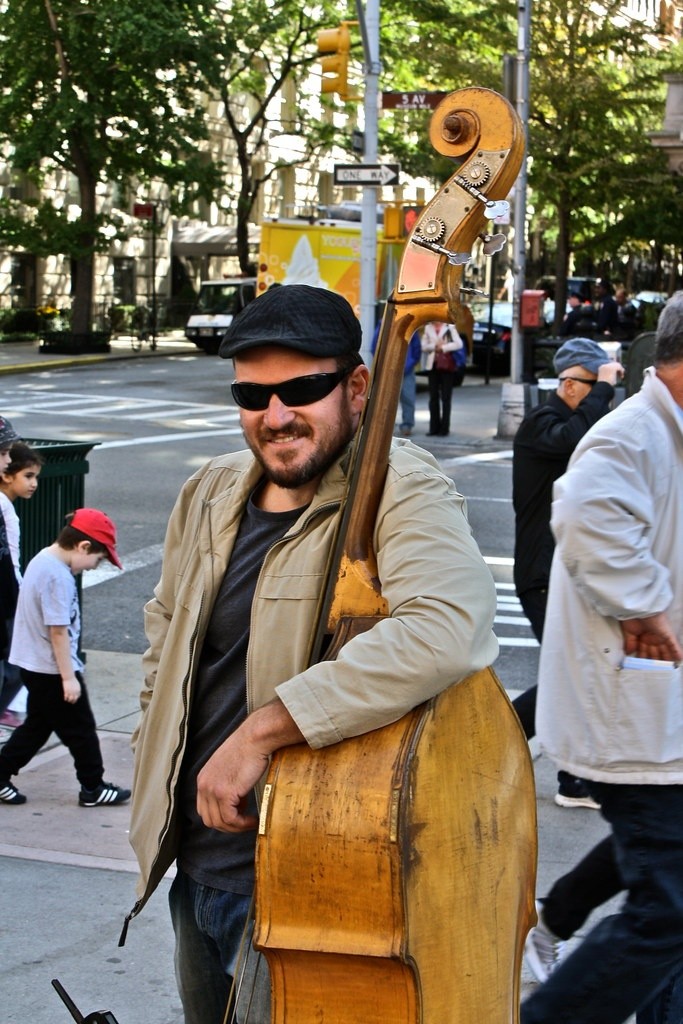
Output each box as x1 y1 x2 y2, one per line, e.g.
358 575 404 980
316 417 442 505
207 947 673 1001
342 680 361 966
333 162 400 187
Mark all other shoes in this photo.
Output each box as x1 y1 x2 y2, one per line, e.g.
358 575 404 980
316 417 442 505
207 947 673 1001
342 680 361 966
0 708 25 727
0 726 11 744
426 430 449 437
401 428 411 436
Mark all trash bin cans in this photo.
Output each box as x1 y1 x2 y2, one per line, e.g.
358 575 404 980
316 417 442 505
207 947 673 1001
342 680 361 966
537 378 560 406
13 436 105 576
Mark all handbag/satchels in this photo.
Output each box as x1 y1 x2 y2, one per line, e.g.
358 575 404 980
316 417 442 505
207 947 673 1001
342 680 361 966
447 323 466 367
437 330 455 369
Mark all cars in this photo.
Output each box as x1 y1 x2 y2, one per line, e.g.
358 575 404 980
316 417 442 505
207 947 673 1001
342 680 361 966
533 276 621 327
631 291 670 317
469 304 513 370
184 278 258 352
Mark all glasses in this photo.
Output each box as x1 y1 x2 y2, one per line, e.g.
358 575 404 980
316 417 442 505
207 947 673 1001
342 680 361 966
559 376 597 390
230 367 354 411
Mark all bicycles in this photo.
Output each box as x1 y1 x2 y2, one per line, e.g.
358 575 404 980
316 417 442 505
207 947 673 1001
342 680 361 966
131 306 156 352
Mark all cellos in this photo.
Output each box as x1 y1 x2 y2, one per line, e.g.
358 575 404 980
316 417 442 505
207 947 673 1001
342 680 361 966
251 87 539 1024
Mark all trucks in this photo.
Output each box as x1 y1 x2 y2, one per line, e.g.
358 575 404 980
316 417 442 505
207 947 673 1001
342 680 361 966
255 201 474 386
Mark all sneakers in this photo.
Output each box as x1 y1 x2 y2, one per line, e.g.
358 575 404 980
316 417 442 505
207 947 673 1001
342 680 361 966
555 769 603 809
78 780 131 807
0 780 27 804
520 896 564 985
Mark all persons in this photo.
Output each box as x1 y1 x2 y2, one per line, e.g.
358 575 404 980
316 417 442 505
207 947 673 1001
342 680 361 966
0 508 131 807
519 291 683 1022
371 319 422 435
563 293 582 337
117 283 500 1024
422 322 463 437
512 337 625 810
498 275 513 302
0 414 44 742
595 290 637 341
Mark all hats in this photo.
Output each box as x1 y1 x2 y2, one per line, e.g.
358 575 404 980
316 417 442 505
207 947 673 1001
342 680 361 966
552 336 611 374
218 284 362 360
69 508 123 570
0 416 21 448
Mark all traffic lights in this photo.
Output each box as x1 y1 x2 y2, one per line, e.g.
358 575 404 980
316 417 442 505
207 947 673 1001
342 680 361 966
318 25 348 95
402 205 427 235
